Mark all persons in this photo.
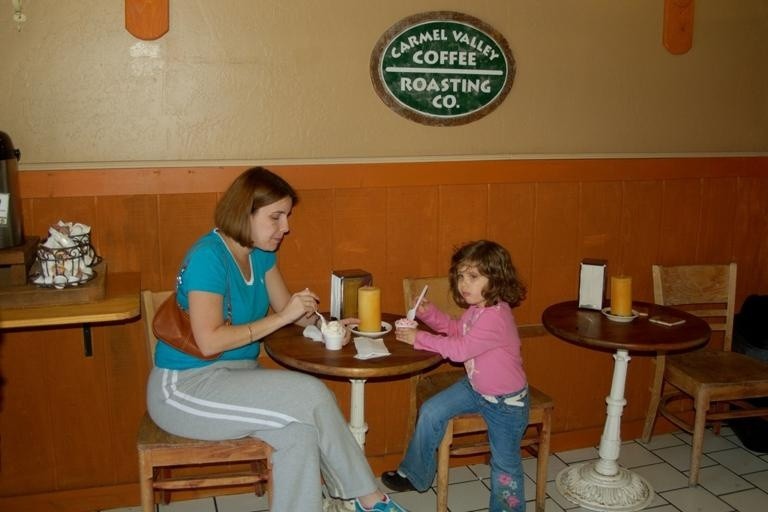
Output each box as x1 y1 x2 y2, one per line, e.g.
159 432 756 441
146 167 411 512
381 240 532 512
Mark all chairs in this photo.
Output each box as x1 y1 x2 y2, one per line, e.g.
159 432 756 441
403 277 556 512
138 289 278 512
643 258 767 487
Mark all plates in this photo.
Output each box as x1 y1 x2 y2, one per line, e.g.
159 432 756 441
600 307 639 324
347 321 392 339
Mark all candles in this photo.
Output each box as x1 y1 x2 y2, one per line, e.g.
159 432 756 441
357 284 382 331
611 276 633 316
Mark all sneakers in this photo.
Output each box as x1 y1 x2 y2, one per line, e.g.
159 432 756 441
381 470 418 492
355 493 407 511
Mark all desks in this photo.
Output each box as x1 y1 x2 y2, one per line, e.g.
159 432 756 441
542 301 710 512
0 270 142 357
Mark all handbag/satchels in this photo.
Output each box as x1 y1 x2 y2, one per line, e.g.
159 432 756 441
151 292 230 360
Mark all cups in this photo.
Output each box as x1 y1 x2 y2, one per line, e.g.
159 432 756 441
322 328 345 351
394 319 417 332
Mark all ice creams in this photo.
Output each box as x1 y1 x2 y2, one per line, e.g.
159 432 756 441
395 318 419 329
323 321 345 351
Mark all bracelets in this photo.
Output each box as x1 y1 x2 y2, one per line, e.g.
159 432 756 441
247 323 253 343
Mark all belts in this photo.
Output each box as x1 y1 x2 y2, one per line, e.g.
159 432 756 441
482 388 527 406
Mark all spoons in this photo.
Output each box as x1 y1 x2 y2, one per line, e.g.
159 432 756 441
305 287 327 330
406 284 428 322
